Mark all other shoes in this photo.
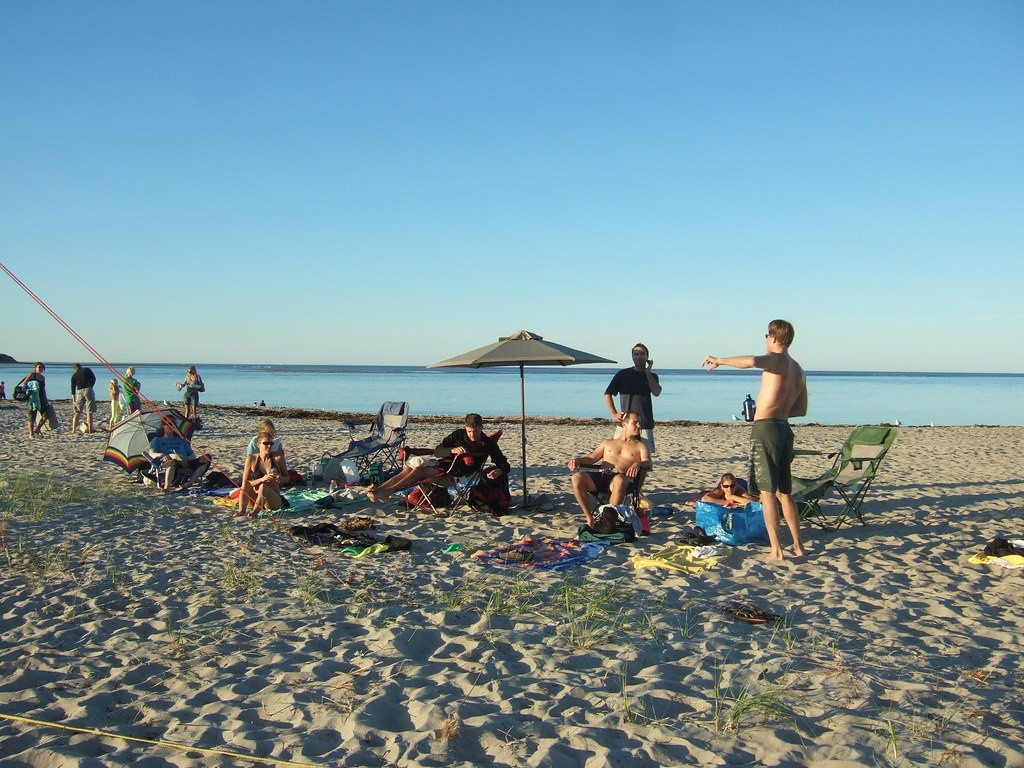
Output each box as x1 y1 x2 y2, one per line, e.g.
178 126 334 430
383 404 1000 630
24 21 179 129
342 517 374 530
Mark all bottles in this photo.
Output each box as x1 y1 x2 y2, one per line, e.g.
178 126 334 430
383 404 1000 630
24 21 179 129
743 394 755 422
329 480 338 495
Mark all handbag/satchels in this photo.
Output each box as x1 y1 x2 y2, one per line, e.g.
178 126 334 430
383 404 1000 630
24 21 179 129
197 375 205 392
13 372 36 400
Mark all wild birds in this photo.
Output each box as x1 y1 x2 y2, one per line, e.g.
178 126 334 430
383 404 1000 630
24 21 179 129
732 414 740 421
163 399 168 405
323 408 325 410
896 419 903 425
931 422 935 427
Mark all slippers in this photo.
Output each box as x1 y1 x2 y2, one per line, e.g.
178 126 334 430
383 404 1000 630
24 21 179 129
377 534 412 553
724 604 782 623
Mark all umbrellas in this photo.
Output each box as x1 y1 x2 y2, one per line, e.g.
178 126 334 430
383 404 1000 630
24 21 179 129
104 407 195 474
427 330 617 505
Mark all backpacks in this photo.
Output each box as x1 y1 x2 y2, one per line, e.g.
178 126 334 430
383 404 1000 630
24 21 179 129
470 467 511 516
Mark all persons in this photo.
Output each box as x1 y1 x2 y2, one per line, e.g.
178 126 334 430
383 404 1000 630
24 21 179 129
254 402 257 406
234 418 289 519
0 381 5 399
702 319 808 560
122 366 140 416
604 343 662 491
70 363 97 433
148 415 212 493
260 400 266 406
22 362 49 440
364 413 511 503
109 379 122 430
568 411 652 528
178 364 204 423
701 473 753 506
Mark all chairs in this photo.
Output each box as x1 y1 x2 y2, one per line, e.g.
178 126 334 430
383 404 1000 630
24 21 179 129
320 401 410 484
141 432 203 489
790 424 903 534
577 432 651 520
396 429 504 517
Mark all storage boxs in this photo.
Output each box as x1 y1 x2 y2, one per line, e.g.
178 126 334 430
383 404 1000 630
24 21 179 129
695 498 768 546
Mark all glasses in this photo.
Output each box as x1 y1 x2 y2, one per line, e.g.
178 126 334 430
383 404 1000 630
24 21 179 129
188 370 193 373
765 334 773 338
259 442 274 445
722 483 735 488
